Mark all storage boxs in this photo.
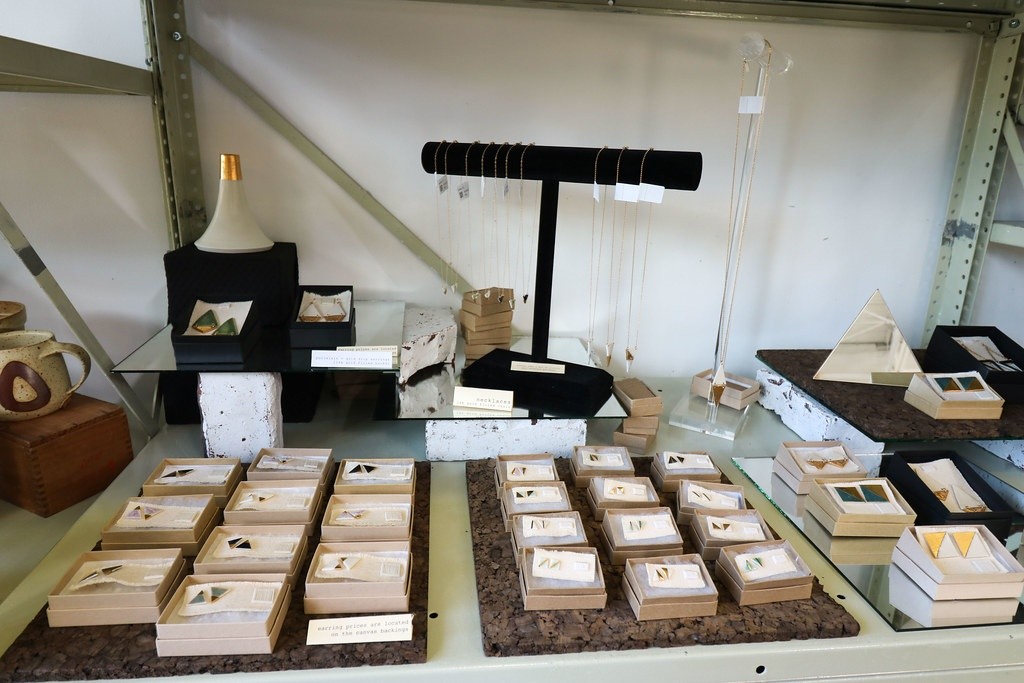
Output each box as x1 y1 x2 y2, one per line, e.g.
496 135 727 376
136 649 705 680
1 392 134 518
45 547 189 628
192 525 309 592
885 449 1015 540
319 494 414 550
921 325 1023 406
903 371 1005 420
613 378 664 457
289 285 358 348
302 542 414 614
691 368 761 411
155 573 292 657
223 478 323 536
333 458 418 504
803 476 917 538
143 457 246 509
650 450 814 606
100 493 221 557
171 293 255 370
491 453 608 611
247 447 335 496
459 287 515 360
570 446 719 621
890 525 1024 601
772 440 868 495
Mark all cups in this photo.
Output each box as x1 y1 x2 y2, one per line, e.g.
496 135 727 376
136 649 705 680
0 301 27 333
0 330 91 421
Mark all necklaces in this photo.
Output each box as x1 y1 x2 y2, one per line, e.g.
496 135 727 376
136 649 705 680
956 339 1023 371
435 140 539 309
713 39 773 407
588 146 654 372
